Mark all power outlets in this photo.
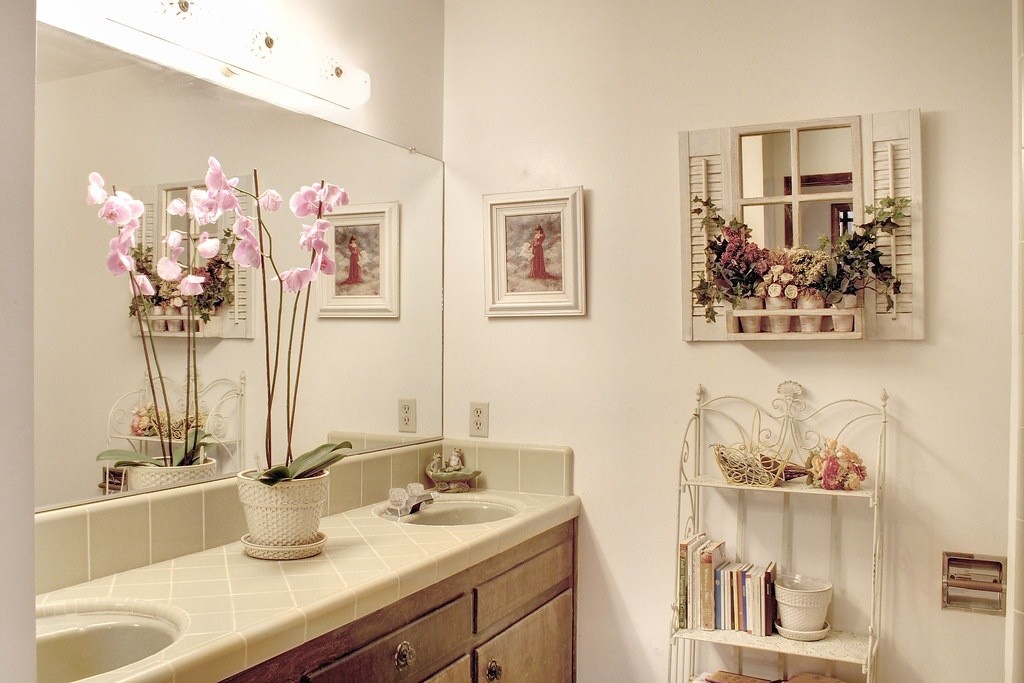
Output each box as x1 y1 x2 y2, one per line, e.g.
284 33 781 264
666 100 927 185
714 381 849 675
396 397 417 435
468 400 490 439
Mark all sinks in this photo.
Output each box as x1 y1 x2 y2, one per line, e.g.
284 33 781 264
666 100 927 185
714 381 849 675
373 497 520 526
33 601 190 683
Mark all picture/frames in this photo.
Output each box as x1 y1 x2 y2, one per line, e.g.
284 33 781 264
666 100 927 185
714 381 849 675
315 199 401 320
482 184 587 318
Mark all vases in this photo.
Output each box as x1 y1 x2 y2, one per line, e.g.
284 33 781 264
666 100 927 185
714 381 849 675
772 574 835 642
766 295 793 334
830 294 857 333
127 455 216 491
142 305 199 334
732 295 763 334
797 296 824 333
236 466 331 561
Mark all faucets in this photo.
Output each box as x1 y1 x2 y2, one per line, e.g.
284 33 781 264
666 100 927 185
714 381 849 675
386 483 434 517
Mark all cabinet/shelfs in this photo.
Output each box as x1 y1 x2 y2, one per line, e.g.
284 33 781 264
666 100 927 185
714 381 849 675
101 368 246 496
666 380 890 683
219 518 577 683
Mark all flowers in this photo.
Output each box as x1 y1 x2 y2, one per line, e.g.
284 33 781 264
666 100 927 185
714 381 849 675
190 155 354 488
805 440 866 492
88 169 217 464
119 227 239 319
680 189 911 321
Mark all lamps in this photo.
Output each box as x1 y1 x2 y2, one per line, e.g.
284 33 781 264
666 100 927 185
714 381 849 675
105 0 370 110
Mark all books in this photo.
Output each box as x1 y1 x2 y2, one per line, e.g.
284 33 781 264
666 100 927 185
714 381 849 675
680 532 776 637
692 671 840 683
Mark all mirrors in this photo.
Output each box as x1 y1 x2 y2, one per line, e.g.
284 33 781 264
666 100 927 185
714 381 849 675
34 18 444 517
